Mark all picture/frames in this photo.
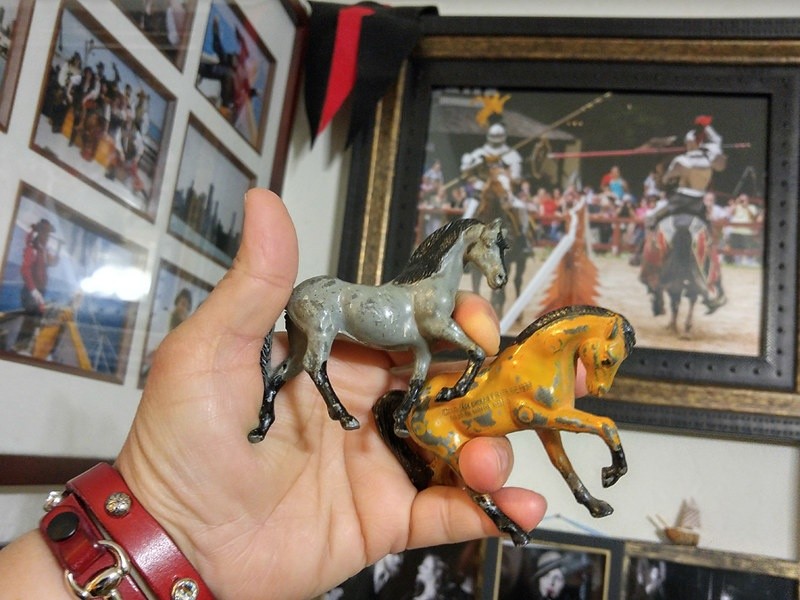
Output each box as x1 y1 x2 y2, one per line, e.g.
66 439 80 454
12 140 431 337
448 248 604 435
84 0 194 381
0 0 313 487
311 528 799 598
334 15 800 448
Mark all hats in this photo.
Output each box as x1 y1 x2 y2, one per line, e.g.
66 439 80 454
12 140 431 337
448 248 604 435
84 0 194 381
32 220 56 232
175 286 192 313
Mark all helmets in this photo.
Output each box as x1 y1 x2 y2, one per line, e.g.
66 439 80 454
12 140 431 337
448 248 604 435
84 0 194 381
488 123 508 144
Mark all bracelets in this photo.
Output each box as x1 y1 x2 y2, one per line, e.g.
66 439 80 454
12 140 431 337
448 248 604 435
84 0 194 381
40 460 218 600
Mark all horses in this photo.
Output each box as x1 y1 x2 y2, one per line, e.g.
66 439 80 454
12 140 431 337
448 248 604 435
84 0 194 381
639 164 721 335
464 154 531 321
371 304 637 546
245 216 510 439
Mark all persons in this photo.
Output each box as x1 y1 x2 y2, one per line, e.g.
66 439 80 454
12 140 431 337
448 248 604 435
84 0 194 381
1 0 257 349
318 554 800 600
1 187 592 600
417 125 763 313
